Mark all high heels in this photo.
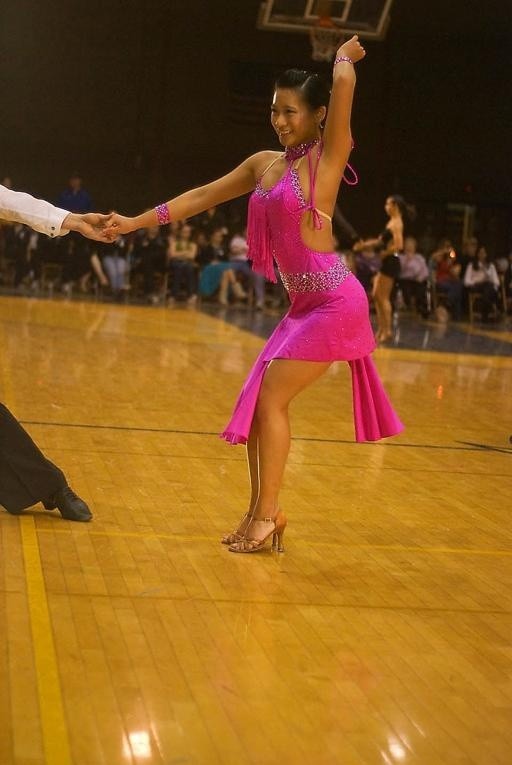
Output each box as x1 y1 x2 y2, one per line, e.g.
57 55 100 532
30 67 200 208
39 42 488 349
220 506 286 554
374 329 394 345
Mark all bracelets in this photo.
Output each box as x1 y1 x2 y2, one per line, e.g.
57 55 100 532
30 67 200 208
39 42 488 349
155 202 170 226
331 56 356 65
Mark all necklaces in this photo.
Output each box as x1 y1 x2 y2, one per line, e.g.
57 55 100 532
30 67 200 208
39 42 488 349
284 137 320 161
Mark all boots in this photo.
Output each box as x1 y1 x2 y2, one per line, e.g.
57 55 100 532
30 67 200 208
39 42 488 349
220 289 229 306
230 280 247 300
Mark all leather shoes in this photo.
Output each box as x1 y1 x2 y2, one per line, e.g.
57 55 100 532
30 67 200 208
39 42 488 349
53 479 93 522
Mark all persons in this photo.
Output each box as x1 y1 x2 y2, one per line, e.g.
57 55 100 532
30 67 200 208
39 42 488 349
354 193 415 345
1 177 282 309
0 185 116 522
102 33 369 552
398 237 501 324
